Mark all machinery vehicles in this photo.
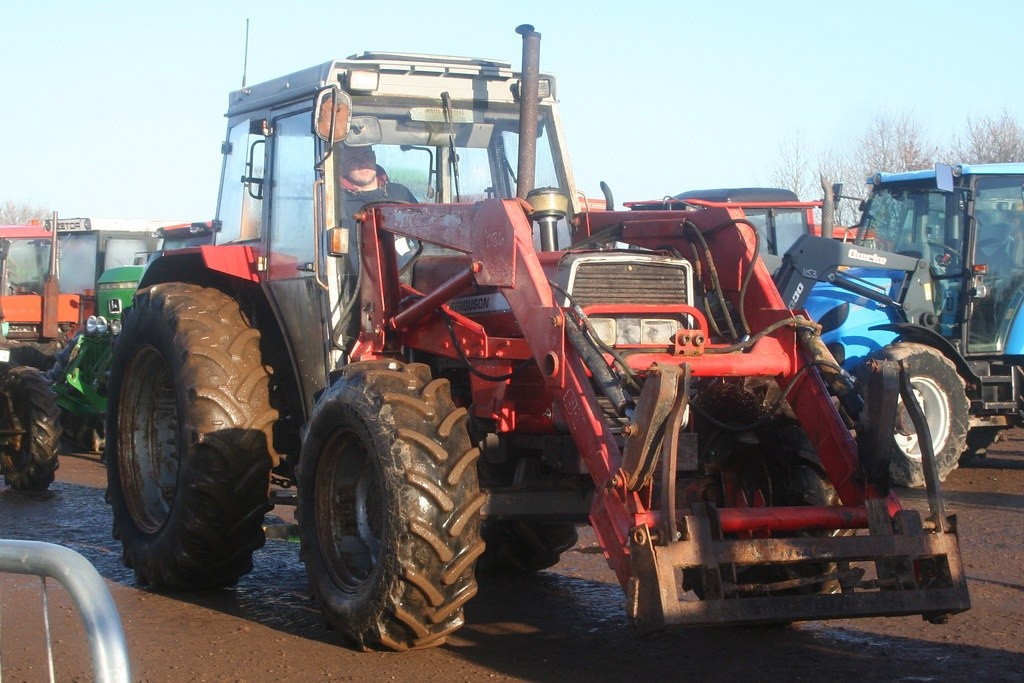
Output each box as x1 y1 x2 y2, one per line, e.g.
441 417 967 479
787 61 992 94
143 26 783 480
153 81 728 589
0 212 213 494
795 163 1024 492
624 187 877 297
100 19 973 656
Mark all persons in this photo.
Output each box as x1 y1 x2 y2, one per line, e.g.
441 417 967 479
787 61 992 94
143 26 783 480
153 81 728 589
337 146 423 281
